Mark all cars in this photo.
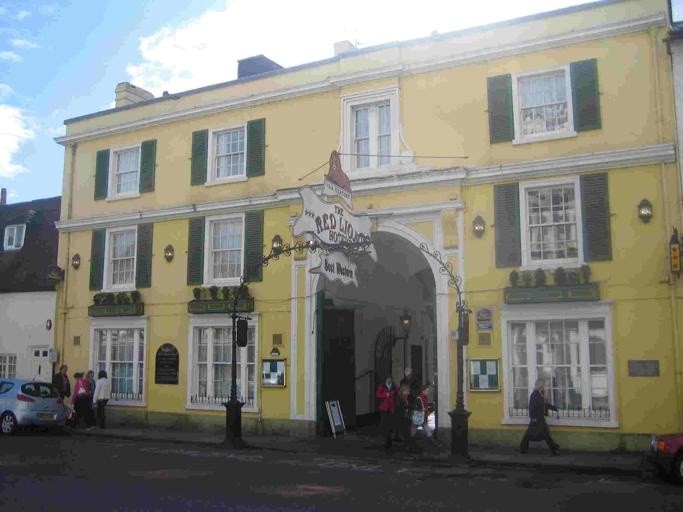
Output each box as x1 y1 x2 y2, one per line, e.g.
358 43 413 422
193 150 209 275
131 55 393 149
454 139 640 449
645 432 682 484
0 378 65 435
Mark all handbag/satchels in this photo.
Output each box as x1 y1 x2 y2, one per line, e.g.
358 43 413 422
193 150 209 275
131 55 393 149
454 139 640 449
78 387 89 399
526 422 547 441
412 410 425 425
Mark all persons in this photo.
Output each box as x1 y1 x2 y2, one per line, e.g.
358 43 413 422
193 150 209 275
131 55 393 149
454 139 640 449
375 372 400 446
519 377 562 453
82 371 96 431
395 384 412 415
53 363 71 400
400 368 420 389
71 370 90 422
409 385 442 453
92 370 110 430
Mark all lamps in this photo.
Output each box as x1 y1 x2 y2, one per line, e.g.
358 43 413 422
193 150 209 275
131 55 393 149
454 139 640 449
639 200 653 222
271 235 282 253
163 244 173 261
472 216 486 237
393 306 411 347
272 345 285 356
72 254 80 270
